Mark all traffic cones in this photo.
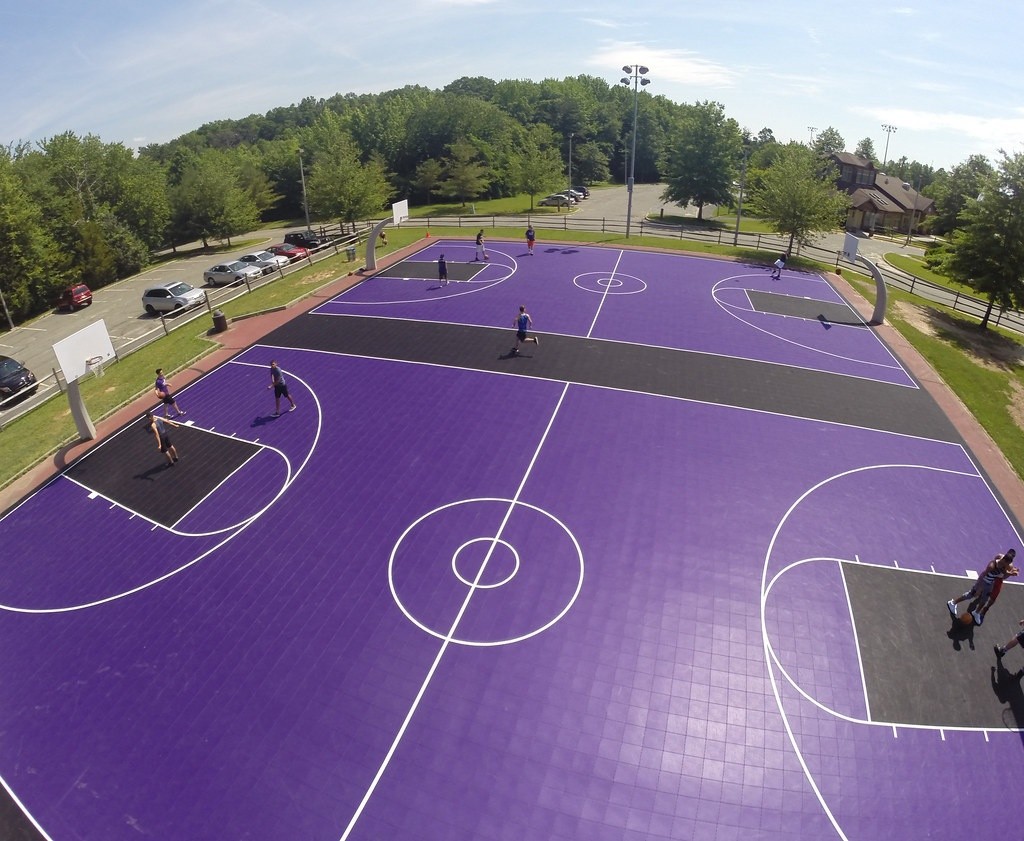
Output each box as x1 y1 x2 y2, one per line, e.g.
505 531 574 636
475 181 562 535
425 228 433 239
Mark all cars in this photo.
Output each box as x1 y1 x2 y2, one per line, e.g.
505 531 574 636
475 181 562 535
264 243 312 262
237 250 292 274
203 260 264 286
543 186 590 202
0 354 39 404
537 194 571 208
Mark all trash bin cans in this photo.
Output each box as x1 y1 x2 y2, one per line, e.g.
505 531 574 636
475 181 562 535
211 309 227 333
345 248 356 261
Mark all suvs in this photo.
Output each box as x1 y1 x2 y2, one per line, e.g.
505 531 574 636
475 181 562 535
282 229 331 249
56 284 93 312
141 281 206 315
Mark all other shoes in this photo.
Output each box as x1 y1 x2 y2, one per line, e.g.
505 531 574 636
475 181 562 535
164 414 174 419
509 348 519 353
288 405 297 412
165 461 175 467
973 598 978 607
534 336 539 346
980 609 986 618
994 643 1006 657
270 414 280 418
178 410 186 416
172 457 179 461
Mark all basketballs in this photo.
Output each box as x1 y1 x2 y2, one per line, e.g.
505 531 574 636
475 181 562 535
158 392 165 399
484 254 488 258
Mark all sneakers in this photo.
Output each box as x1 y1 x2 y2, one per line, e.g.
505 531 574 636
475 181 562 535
947 598 958 615
972 609 981 626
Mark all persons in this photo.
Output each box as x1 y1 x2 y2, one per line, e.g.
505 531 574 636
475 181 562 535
379 231 387 246
154 368 187 419
993 619 1024 659
947 549 1020 625
511 305 539 353
438 253 449 288
146 412 179 466
525 225 536 254
772 247 791 279
475 229 490 261
266 360 297 417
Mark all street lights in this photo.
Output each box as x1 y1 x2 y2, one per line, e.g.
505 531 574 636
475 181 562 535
567 130 576 213
730 141 751 246
620 62 651 238
902 173 924 243
294 146 315 231
808 126 820 157
882 124 900 172
